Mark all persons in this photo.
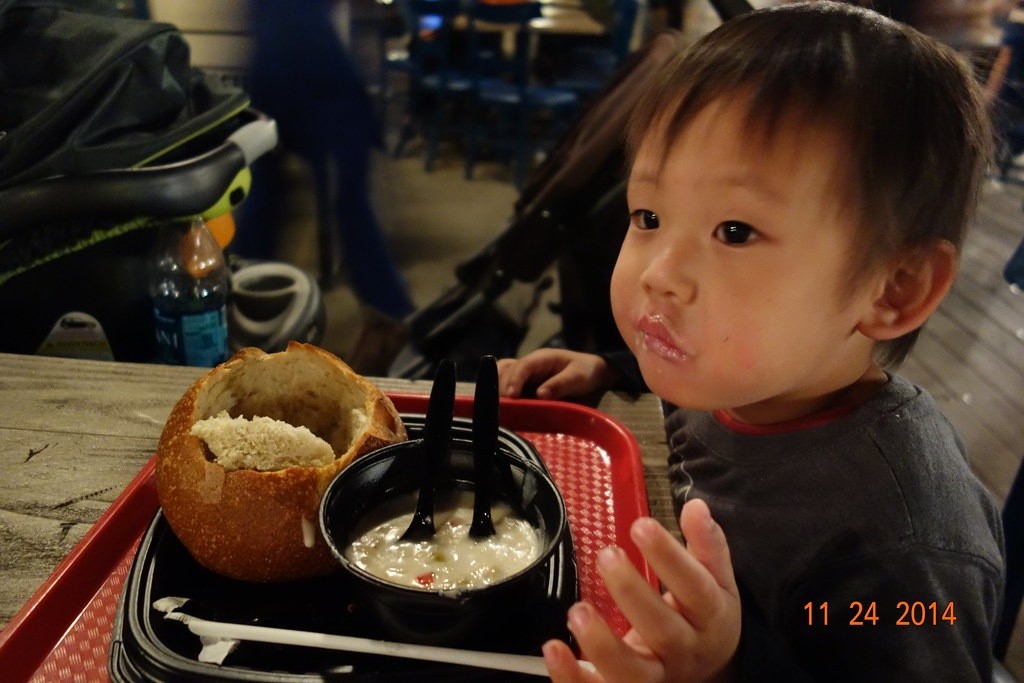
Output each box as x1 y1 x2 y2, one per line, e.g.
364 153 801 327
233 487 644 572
494 0 1005 683
983 0 1024 683
231 0 758 356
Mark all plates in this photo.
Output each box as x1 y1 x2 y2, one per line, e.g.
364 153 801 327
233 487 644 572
107 409 585 682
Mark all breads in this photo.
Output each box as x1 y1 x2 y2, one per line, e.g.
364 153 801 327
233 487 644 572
154 339 410 582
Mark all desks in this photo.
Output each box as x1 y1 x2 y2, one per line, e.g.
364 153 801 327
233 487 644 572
452 0 604 66
0 351 686 683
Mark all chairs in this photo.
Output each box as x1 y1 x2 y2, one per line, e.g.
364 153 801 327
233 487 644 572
373 0 651 196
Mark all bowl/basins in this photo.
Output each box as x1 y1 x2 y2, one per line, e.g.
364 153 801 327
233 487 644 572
320 438 571 614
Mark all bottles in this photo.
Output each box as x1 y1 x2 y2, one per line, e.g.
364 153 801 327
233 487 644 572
147 214 234 369
